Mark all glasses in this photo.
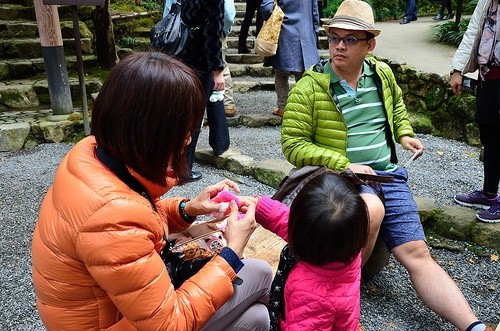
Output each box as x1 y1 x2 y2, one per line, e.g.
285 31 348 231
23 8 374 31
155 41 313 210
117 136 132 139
325 32 368 45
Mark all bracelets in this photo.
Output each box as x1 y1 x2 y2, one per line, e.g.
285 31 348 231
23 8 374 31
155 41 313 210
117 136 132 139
181 198 197 222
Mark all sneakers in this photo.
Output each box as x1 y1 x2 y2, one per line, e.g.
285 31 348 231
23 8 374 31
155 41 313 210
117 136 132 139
454 189 500 209
218 146 241 158
475 203 500 223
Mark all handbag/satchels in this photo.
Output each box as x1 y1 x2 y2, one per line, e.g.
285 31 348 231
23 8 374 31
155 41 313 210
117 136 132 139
150 0 201 58
271 165 394 282
254 0 286 57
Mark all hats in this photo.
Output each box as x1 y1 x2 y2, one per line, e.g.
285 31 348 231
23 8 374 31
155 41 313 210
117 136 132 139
322 0 381 37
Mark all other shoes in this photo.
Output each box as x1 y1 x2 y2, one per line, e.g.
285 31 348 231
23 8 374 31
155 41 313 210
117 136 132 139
400 19 410 24
272 106 286 117
432 13 443 21
443 12 454 20
238 50 251 53
225 103 237 117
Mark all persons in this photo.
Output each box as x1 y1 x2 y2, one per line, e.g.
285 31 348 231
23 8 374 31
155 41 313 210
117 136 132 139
432 0 454 20
235 172 370 331
32 52 273 331
238 0 263 54
260 0 320 117
281 14 495 331
180 0 230 182
451 0 500 223
163 0 236 126
400 0 417 24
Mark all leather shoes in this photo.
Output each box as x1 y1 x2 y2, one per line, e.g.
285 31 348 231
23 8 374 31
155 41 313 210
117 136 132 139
187 169 204 182
